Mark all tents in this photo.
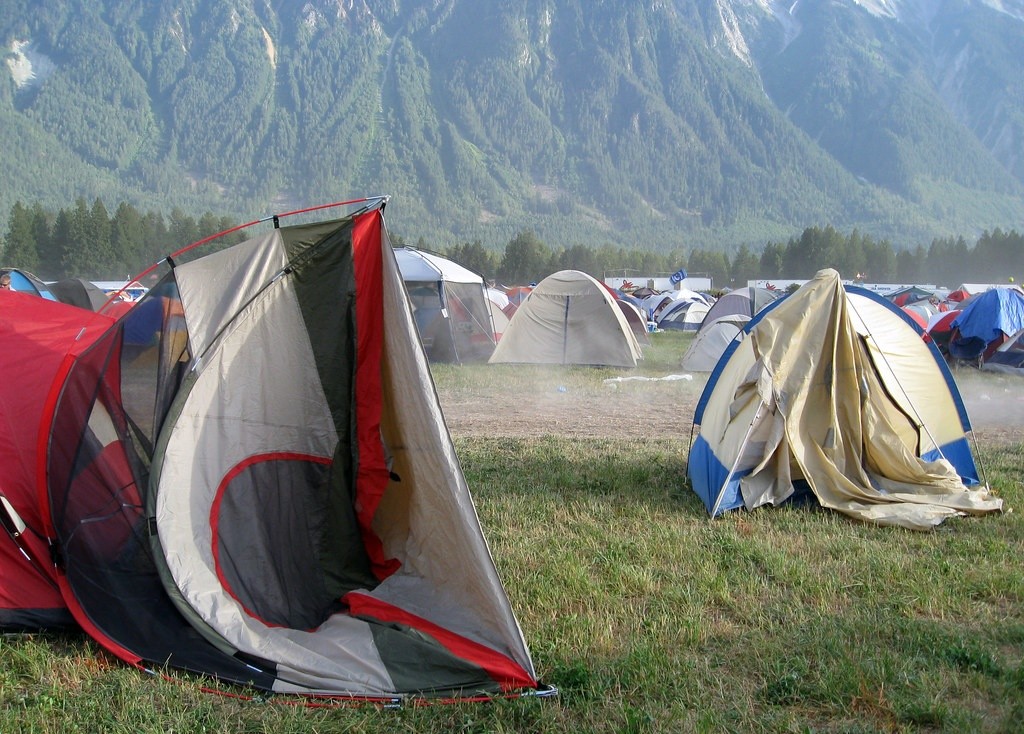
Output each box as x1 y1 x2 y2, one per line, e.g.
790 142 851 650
684 268 1003 531
0 195 1024 708
